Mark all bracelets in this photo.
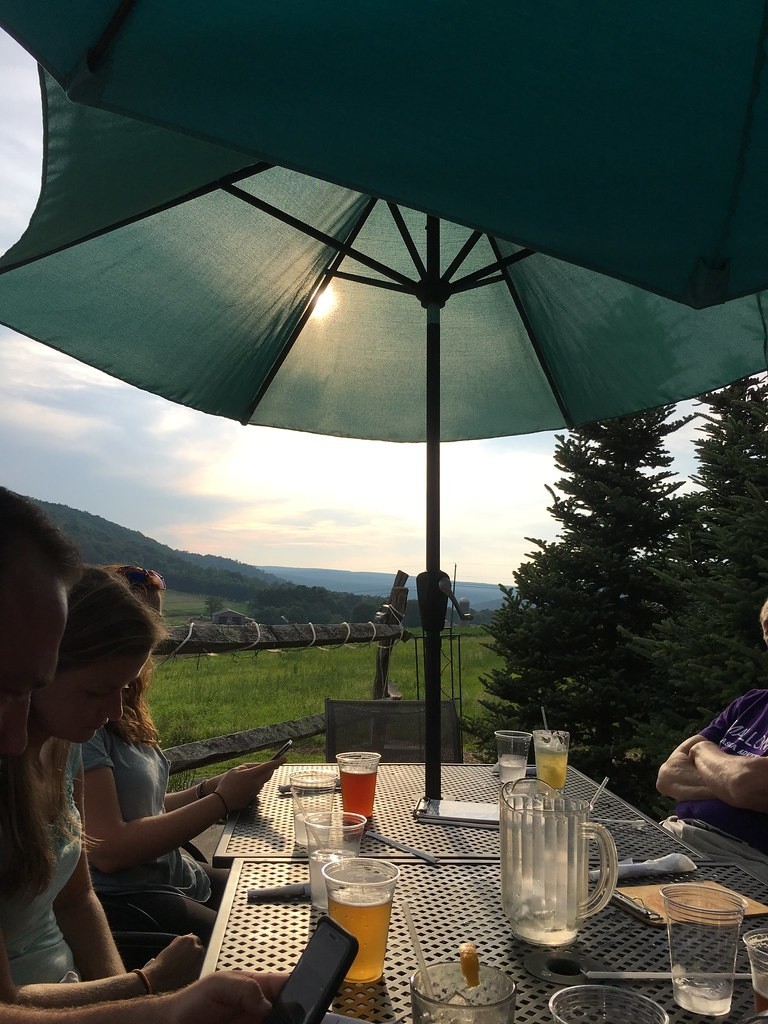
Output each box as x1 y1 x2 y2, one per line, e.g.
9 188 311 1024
212 790 229 824
131 969 152 996
195 779 205 799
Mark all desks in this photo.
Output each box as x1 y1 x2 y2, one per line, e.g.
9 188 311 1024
200 763 768 1024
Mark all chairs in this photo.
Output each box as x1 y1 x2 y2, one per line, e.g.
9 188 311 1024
324 697 462 766
95 841 209 948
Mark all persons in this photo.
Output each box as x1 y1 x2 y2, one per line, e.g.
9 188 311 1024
654 602 768 883
1 486 292 1024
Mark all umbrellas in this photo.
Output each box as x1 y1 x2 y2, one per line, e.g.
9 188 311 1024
0 1 767 802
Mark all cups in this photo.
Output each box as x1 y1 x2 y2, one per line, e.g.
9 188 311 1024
494 730 533 784
548 984 669 1024
409 961 517 1024
289 769 340 846
321 858 400 984
303 812 367 911
532 730 570 796
336 751 381 817
659 884 749 1017
742 928 768 1014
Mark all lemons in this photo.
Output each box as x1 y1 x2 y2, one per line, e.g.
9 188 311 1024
458 942 481 988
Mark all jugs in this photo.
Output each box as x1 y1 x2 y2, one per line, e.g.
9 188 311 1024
499 777 619 947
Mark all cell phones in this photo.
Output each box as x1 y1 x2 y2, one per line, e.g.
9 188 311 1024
271 740 293 759
267 915 358 1024
